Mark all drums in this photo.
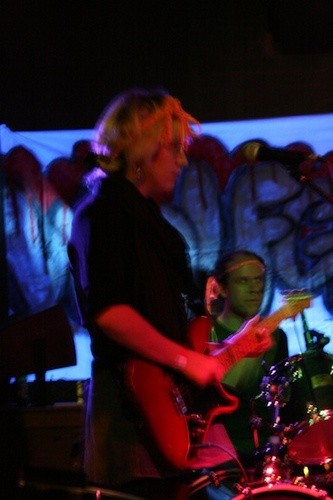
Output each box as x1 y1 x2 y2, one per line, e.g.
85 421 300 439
54 487 140 500
253 349 333 467
176 466 332 500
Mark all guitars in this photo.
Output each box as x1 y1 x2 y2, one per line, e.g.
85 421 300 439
116 288 313 473
